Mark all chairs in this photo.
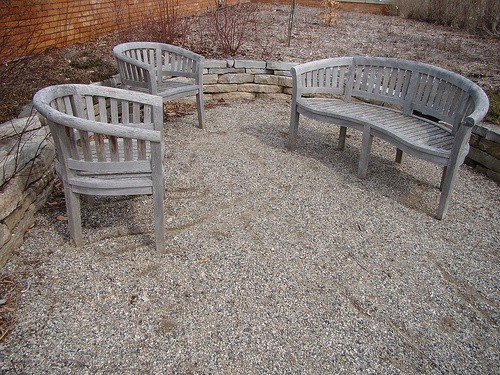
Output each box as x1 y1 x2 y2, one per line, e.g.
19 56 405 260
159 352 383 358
112 41 206 130
33 84 167 254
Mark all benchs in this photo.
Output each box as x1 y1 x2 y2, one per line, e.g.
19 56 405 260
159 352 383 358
289 55 490 220
